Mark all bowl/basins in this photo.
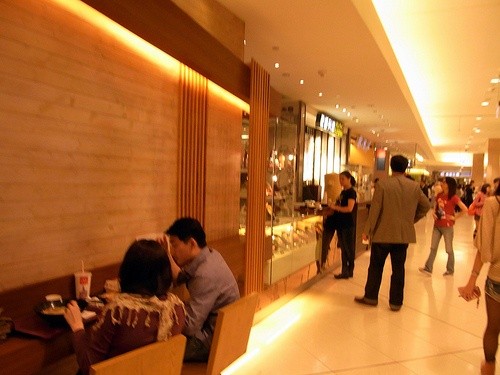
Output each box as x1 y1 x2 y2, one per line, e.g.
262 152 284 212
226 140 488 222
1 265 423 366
36 297 85 326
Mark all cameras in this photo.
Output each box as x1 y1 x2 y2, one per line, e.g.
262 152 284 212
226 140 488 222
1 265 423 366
435 210 441 219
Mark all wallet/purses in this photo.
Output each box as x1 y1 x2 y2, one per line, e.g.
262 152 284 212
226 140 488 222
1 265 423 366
457 285 481 308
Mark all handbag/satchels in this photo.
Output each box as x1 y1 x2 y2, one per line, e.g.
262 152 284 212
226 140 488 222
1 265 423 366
468 197 479 216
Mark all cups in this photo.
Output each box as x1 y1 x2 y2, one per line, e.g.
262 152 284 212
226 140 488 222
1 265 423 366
74 272 91 299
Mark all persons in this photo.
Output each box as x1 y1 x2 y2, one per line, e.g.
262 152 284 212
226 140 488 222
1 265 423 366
367 177 380 212
419 176 467 275
326 171 356 280
64 239 187 375
157 216 240 362
354 155 430 311
405 155 500 375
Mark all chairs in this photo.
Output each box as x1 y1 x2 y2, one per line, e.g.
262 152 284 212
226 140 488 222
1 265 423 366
88 332 187 375
182 291 258 375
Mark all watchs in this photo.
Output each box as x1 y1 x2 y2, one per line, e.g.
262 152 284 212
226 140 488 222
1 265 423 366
471 268 480 278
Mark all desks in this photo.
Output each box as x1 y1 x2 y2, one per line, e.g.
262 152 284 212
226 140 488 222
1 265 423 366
15 297 108 339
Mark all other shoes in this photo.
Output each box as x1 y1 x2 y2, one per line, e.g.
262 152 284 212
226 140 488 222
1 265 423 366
419 266 432 276
389 299 401 311
355 296 378 306
334 273 353 279
